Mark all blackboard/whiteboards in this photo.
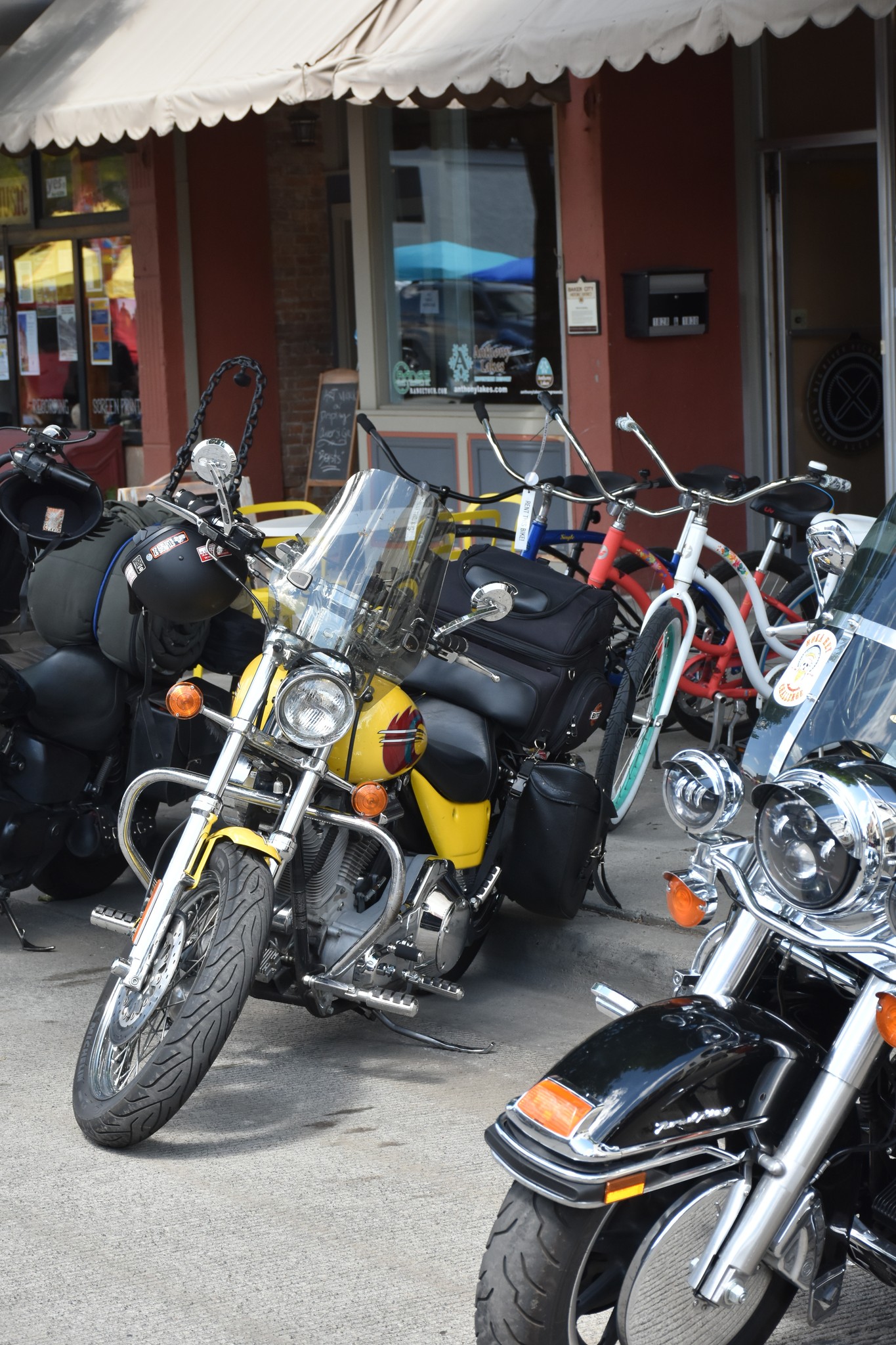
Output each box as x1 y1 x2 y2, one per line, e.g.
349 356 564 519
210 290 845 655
306 369 361 487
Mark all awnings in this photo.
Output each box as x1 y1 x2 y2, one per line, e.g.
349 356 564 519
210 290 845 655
0 0 895 151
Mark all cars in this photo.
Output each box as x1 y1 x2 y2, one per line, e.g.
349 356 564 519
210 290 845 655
389 277 535 406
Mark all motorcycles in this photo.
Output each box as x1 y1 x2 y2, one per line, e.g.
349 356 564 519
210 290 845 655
72 435 626 1155
0 356 270 951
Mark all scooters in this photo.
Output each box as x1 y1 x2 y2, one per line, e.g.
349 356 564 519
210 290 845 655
472 479 896 1345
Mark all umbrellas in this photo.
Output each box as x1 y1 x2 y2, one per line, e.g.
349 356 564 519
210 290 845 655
398 278 520 331
1 236 139 365
391 238 533 286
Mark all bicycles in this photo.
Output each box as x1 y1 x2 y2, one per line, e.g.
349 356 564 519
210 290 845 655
356 387 895 835
